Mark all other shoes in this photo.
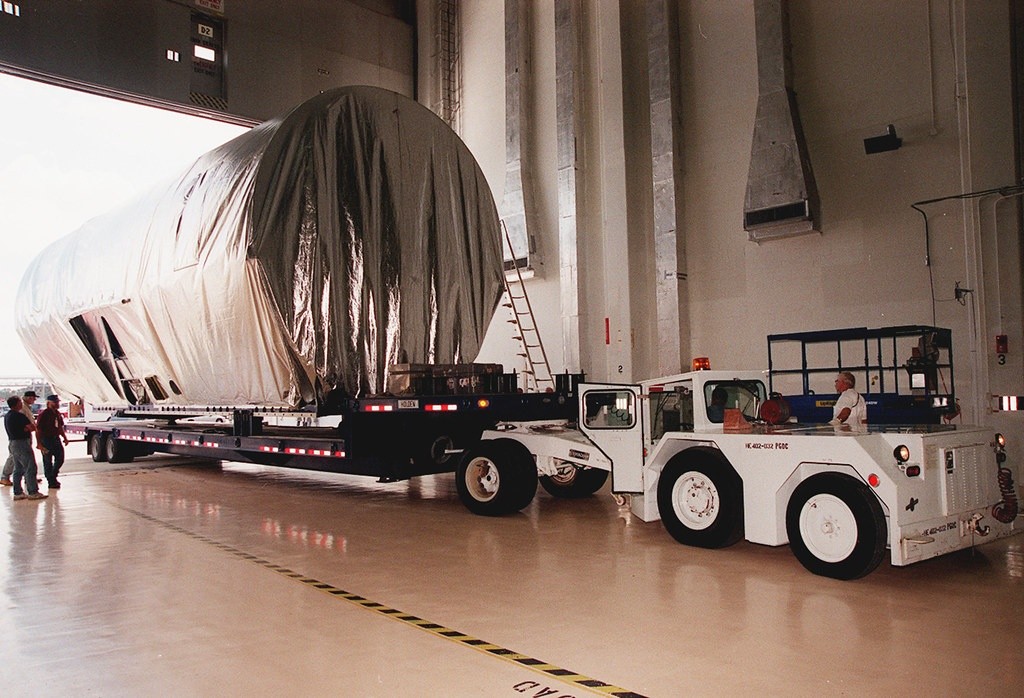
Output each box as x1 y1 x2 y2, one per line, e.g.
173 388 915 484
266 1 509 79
49 483 59 488
25 479 42 483
0 479 13 486
14 493 28 499
28 492 50 499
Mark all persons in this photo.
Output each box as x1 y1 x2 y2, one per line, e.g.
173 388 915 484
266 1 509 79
36 395 68 488
833 372 867 424
0 391 42 486
4 396 49 500
707 388 756 422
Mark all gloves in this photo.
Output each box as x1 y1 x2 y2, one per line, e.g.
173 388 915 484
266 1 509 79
828 418 842 425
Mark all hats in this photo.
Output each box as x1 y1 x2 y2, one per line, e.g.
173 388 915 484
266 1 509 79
25 391 39 397
47 395 60 402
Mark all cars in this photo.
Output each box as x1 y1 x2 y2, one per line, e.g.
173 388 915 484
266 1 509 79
0 400 83 420
